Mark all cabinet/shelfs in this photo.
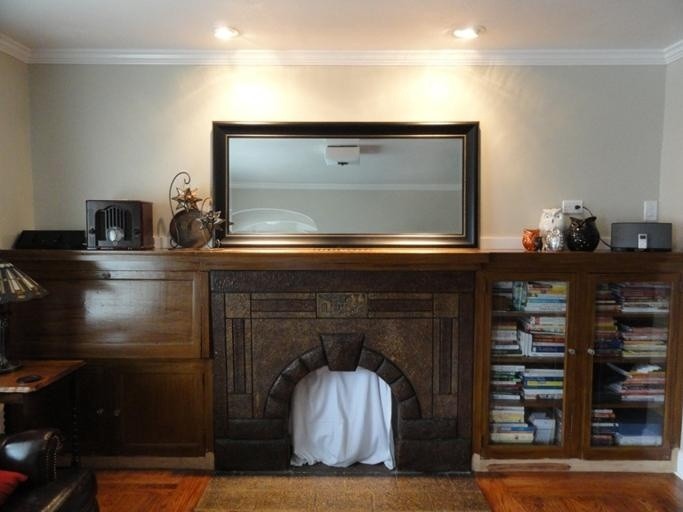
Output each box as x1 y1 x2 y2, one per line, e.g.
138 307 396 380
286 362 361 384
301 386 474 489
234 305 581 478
0 255 213 477
480 253 682 463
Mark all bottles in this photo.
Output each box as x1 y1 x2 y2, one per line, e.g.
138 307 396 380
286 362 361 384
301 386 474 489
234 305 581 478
522 198 599 251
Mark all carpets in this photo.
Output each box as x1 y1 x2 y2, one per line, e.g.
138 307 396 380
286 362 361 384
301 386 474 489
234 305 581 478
188 471 490 511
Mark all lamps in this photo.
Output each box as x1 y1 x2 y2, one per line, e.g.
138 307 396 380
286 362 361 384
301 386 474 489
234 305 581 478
0 262 49 373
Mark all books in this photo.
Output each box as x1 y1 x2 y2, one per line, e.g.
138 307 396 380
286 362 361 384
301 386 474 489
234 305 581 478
490 272 666 450
